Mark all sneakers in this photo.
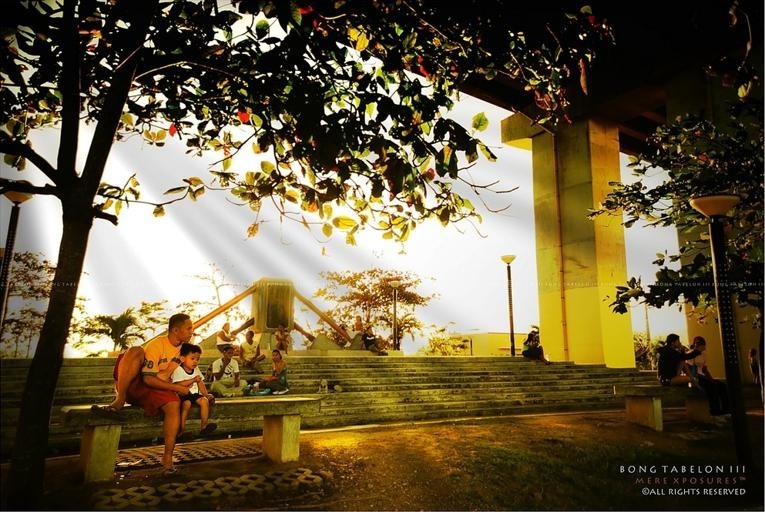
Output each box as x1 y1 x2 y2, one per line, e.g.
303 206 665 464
273 389 289 395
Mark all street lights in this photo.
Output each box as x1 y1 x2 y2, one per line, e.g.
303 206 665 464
690 192 747 442
0 181 34 331
500 255 517 354
389 281 399 347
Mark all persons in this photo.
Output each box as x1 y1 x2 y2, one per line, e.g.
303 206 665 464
334 315 387 355
642 334 730 416
170 343 217 439
211 322 289 396
521 333 550 365
747 348 760 386
91 313 194 476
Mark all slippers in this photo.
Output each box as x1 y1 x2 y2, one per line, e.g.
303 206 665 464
177 430 193 442
91 404 127 422
200 422 217 434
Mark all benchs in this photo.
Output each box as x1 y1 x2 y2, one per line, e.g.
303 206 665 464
60 397 320 484
614 378 727 432
